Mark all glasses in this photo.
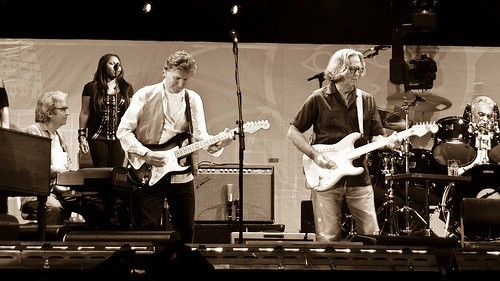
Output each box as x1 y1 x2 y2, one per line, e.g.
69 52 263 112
54 107 68 112
347 68 364 74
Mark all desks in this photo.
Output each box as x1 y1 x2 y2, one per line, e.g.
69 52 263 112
392 173 471 240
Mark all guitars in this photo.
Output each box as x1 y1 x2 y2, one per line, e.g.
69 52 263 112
126 119 271 187
302 119 439 192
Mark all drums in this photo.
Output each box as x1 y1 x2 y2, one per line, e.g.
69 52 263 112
440 163 500 241
368 148 406 204
486 119 500 163
394 147 447 204
432 116 478 167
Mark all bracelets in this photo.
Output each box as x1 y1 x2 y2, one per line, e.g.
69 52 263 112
78 134 86 142
215 144 221 150
78 128 86 133
385 145 387 149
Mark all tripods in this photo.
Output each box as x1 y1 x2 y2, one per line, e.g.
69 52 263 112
307 52 430 241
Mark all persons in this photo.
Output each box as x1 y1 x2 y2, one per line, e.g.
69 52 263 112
23 91 73 223
287 49 403 242
448 96 500 176
116 50 234 244
78 53 134 167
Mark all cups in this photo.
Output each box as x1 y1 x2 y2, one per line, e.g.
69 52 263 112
448 160 459 176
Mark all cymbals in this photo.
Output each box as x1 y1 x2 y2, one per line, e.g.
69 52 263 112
378 109 410 130
386 91 452 113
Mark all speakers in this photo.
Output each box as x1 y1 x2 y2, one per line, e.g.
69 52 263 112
462 198 500 241
0 126 51 197
195 164 275 224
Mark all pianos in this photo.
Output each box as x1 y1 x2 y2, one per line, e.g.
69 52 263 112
56 167 131 225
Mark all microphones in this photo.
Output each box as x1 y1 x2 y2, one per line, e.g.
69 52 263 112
226 184 234 220
370 45 391 50
113 63 119 70
230 30 238 42
